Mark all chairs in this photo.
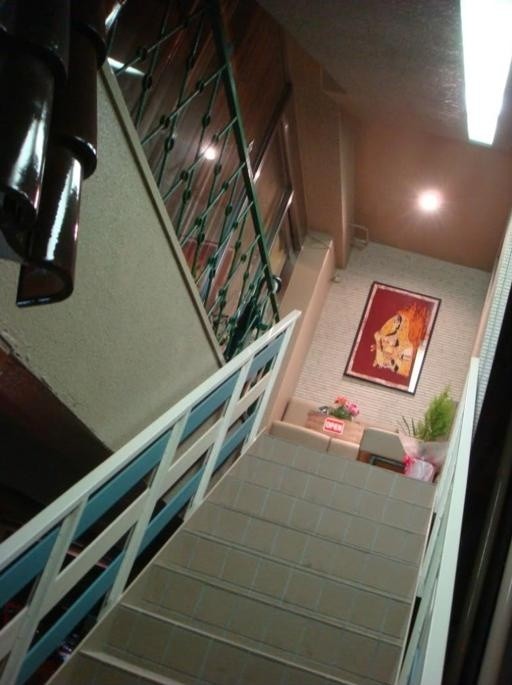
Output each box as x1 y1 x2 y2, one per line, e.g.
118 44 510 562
360 425 425 473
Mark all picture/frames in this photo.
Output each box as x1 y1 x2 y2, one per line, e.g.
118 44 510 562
178 234 235 316
344 281 441 395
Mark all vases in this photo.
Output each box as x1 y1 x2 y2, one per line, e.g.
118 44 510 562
329 406 352 420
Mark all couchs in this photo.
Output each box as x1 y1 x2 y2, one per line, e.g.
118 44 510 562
268 398 414 459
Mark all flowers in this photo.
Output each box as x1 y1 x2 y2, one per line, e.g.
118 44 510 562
335 395 359 416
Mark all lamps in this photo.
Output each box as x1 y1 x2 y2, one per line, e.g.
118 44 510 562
459 0 512 145
